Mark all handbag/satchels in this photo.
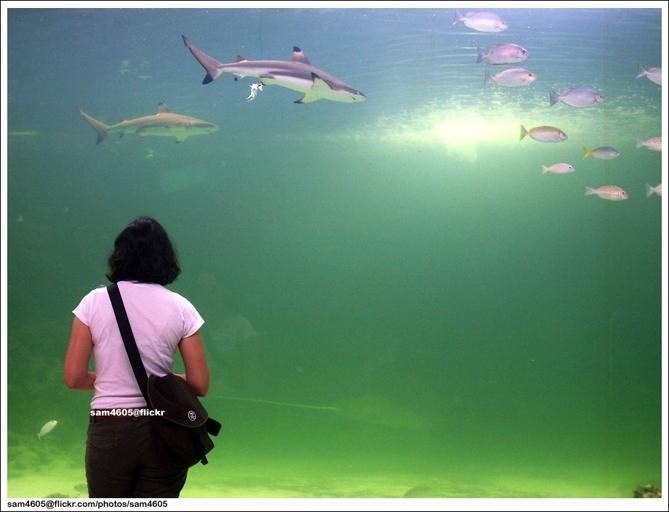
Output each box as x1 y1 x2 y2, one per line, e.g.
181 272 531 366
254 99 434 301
146 372 221 470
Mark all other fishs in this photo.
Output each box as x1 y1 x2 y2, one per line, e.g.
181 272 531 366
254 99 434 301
580 144 623 162
546 85 607 110
635 60 663 87
633 135 663 153
583 183 629 202
36 418 59 440
540 162 577 176
644 179 663 199
450 10 510 34
517 122 570 145
470 39 539 88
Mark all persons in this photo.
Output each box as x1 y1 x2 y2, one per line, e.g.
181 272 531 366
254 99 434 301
64 215 209 499
